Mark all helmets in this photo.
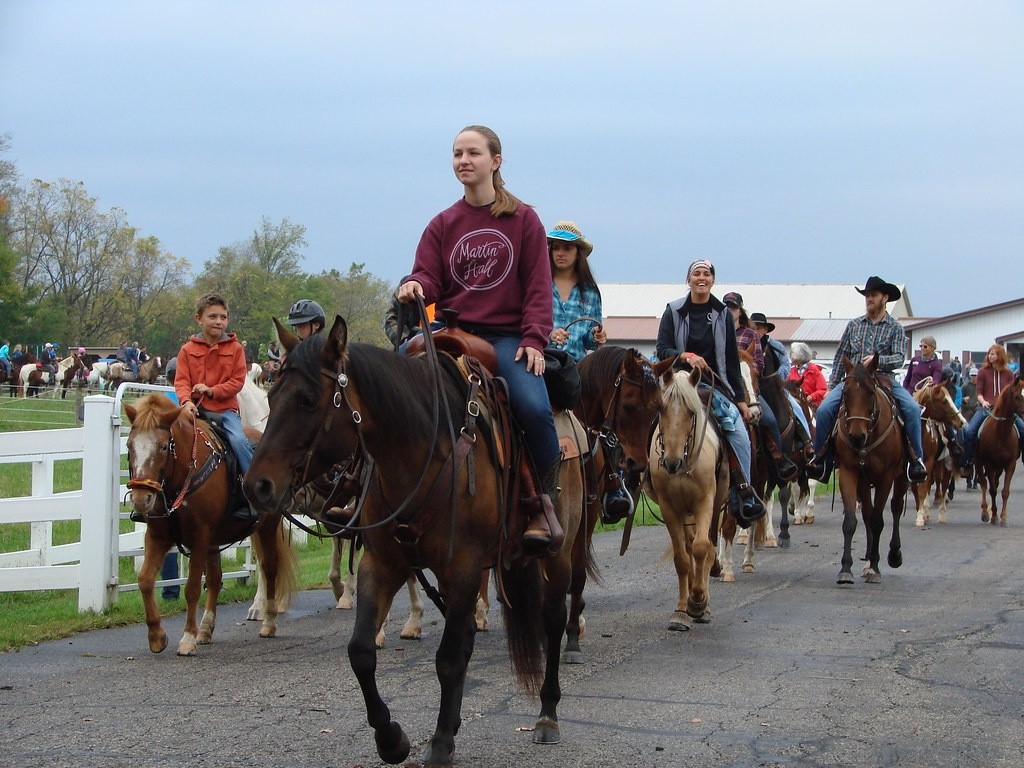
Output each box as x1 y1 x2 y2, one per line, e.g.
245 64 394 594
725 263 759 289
284 298 326 332
941 367 954 378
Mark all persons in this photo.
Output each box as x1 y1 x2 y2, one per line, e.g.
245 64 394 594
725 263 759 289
323 125 562 545
285 276 434 354
545 221 631 524
175 294 256 521
656 259 1024 529
0 340 285 601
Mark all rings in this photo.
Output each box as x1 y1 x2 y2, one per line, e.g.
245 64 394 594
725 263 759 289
556 336 560 340
536 357 544 361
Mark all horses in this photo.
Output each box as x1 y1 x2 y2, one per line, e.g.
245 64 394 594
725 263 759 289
14 354 87 399
120 393 302 657
28 354 93 399
531 345 680 662
647 361 733 633
0 352 37 399
255 351 286 387
717 336 1024 585
242 313 585 768
302 430 491 648
102 354 162 395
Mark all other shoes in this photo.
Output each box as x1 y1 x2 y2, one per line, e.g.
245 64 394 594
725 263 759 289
780 460 796 477
962 467 972 478
523 510 550 545
132 511 145 522
230 504 260 520
604 495 630 525
909 465 927 480
806 462 830 484
952 445 960 455
735 504 762 529
329 507 358 528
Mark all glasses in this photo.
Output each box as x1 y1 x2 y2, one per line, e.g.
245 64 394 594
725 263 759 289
920 345 929 348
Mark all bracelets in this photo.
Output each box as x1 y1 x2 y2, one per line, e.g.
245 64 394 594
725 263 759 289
687 352 694 362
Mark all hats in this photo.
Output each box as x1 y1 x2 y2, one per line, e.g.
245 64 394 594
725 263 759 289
545 221 593 259
969 368 979 375
723 292 744 307
854 276 901 303
45 343 54 349
78 348 86 353
750 313 775 333
